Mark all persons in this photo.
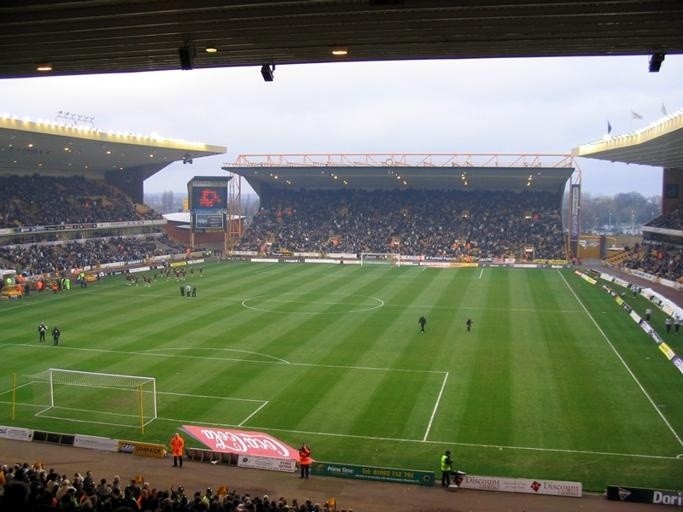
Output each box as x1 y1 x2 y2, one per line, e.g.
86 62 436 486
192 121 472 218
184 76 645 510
212 184 586 267
418 315 426 332
51 326 60 345
616 206 683 333
0 172 207 300
1 459 338 511
440 449 453 487
298 440 312 480
169 431 185 466
37 321 47 342
465 318 474 332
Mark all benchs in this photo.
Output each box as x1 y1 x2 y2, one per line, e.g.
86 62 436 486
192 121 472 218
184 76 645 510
0 178 163 228
601 242 683 284
0 225 179 274
